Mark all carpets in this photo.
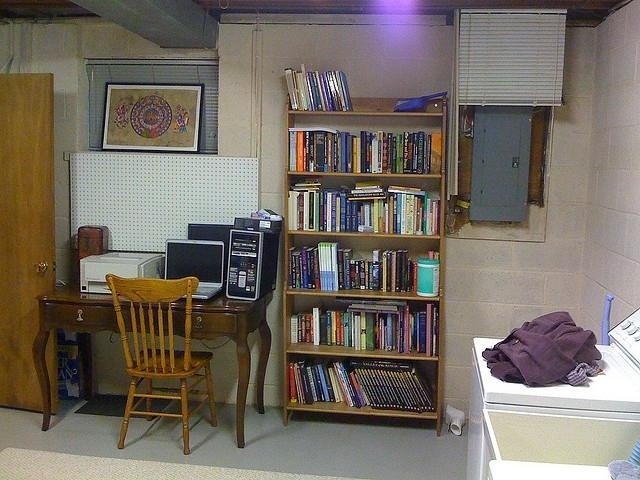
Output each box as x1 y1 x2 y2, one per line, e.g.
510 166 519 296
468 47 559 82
0 448 347 480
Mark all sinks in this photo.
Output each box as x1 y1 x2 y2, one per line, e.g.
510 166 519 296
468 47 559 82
480 407 640 473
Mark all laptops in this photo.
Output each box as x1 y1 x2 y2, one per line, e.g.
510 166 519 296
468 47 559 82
165 239 224 300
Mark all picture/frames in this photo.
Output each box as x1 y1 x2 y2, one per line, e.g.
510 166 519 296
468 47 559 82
102 83 204 153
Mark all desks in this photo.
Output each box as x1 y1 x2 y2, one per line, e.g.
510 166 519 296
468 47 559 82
33 286 271 448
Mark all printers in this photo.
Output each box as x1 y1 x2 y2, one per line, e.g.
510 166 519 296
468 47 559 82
80 252 164 294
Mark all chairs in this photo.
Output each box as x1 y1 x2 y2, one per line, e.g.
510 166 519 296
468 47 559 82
106 273 217 455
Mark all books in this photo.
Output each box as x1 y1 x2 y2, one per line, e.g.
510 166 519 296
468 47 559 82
289 128 442 174
284 64 353 112
290 297 438 355
289 360 434 413
288 178 440 235
289 242 439 292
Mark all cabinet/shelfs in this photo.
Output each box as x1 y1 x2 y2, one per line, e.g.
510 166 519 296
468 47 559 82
282 98 449 436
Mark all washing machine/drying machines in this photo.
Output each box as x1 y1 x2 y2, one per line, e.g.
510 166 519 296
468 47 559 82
465 306 640 480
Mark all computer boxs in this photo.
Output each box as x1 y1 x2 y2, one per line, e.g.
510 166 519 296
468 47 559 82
226 229 280 301
